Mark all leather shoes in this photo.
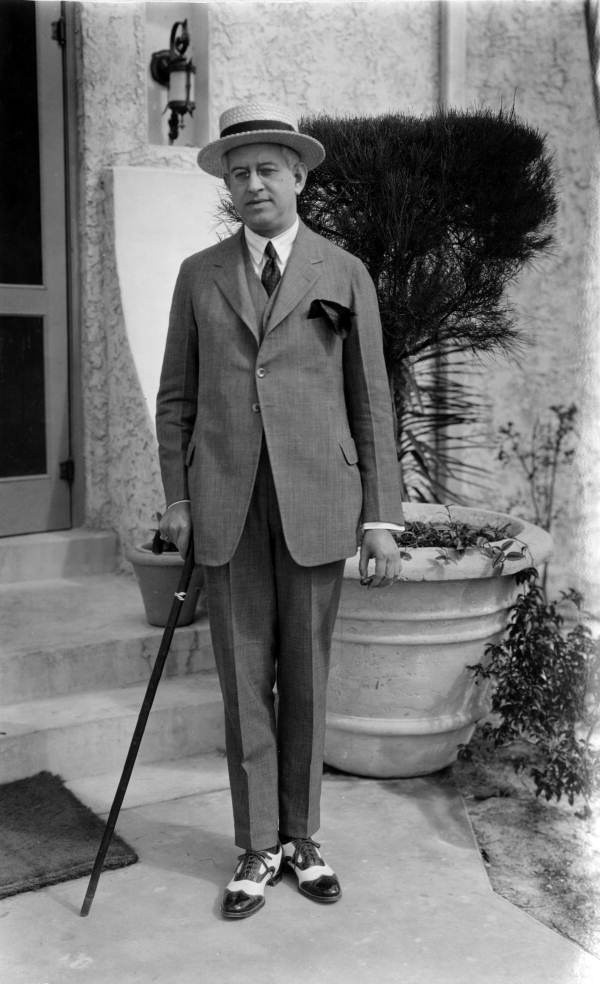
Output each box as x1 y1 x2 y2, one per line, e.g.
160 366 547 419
282 833 340 903
220 841 290 919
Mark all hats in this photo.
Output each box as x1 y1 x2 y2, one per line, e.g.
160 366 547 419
197 105 325 177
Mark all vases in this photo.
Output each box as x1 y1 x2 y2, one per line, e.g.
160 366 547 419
126 544 202 627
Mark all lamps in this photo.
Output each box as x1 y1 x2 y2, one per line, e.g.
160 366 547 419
150 18 199 142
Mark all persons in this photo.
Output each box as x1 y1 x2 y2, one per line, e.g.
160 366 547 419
155 101 407 918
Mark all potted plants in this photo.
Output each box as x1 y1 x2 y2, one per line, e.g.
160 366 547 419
252 106 554 778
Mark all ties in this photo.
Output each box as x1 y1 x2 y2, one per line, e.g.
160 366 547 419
261 241 285 297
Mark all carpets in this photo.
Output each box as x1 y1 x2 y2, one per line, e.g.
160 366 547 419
0 770 138 899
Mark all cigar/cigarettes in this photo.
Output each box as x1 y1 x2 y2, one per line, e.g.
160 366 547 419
360 574 377 586
